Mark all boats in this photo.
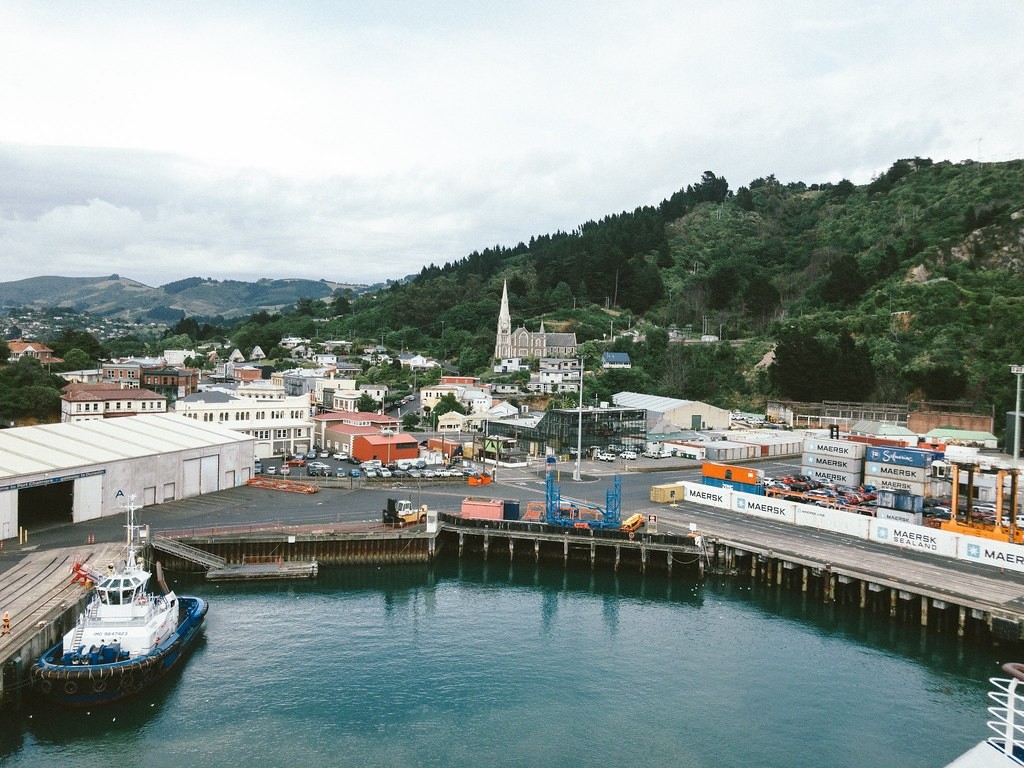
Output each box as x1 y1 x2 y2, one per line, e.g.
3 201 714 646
27 488 210 705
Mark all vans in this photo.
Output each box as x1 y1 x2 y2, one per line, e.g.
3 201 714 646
597 453 616 462
620 451 637 460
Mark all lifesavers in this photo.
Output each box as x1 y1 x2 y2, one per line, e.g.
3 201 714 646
142 669 154 682
158 661 167 675
628 532 635 539
41 680 51 694
120 675 134 688
64 681 77 694
138 597 146 604
27 676 34 689
92 680 107 693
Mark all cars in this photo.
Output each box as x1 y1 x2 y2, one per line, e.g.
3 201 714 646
319 450 330 458
295 453 305 461
332 451 348 461
568 444 673 459
305 457 475 478
306 449 318 460
267 466 277 474
281 464 290 474
285 459 306 468
759 473 1024 531
254 463 263 474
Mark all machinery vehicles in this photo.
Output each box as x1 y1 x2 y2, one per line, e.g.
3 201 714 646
382 497 428 528
